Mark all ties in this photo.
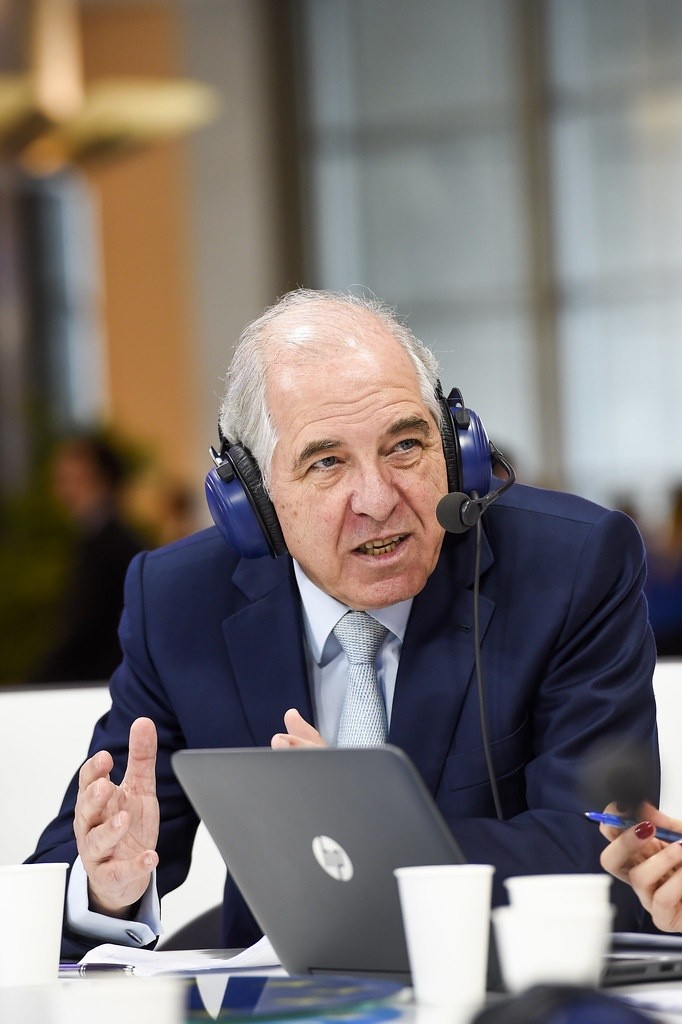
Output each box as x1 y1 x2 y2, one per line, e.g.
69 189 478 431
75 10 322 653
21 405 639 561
335 608 390 750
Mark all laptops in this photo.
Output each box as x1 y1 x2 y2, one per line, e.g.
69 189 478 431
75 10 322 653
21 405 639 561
170 744 682 988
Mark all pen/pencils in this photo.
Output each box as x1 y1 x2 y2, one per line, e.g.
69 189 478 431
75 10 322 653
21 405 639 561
582 811 682 846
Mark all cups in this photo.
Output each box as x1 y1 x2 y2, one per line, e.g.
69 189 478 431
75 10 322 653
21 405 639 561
489 905 618 995
503 872 615 908
391 861 494 1024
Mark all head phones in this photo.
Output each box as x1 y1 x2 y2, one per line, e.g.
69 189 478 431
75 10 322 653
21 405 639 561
201 377 492 561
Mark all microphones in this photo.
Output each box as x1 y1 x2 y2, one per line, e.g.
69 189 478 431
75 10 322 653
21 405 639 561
435 440 517 534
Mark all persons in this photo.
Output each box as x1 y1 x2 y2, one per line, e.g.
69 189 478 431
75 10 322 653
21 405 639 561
21 288 661 968
43 428 162 687
487 450 681 661
598 796 682 937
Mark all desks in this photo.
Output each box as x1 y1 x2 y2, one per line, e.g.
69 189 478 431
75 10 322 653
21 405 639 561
7 947 680 1024
